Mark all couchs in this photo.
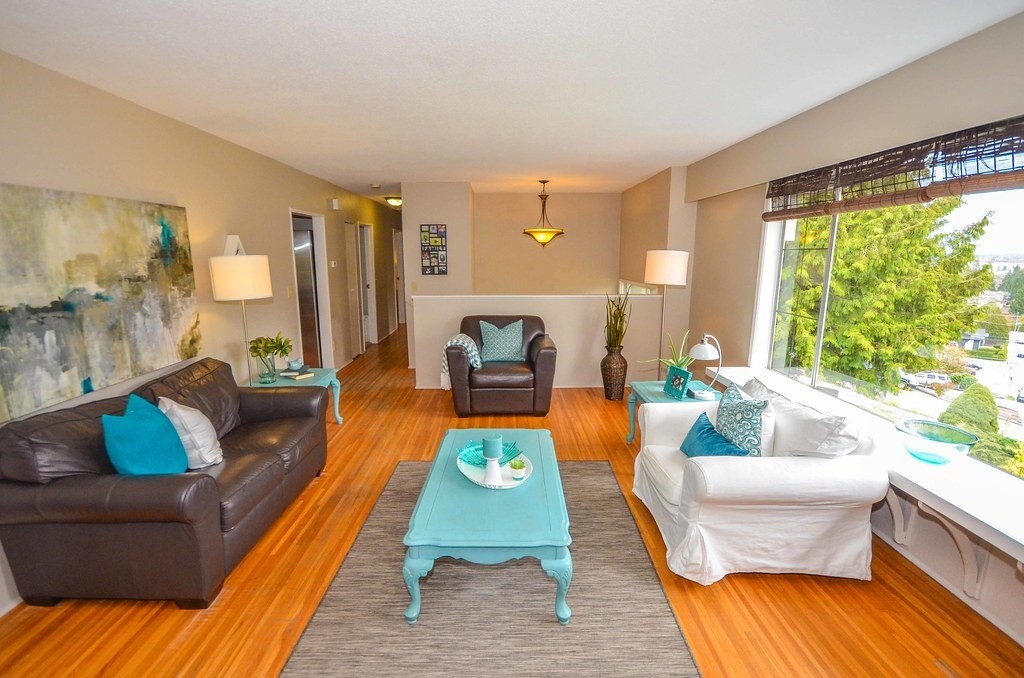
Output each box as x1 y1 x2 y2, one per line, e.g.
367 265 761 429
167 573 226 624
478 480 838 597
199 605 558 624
446 315 557 420
631 376 889 586
0 357 329 609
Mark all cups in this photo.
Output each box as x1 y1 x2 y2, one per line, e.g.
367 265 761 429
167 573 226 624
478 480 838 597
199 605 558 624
510 466 526 481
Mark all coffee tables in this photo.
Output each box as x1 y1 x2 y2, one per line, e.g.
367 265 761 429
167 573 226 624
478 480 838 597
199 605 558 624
626 379 723 442
243 368 344 425
401 428 574 627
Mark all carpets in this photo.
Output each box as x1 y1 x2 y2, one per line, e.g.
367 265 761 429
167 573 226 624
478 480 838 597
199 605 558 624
278 458 701 678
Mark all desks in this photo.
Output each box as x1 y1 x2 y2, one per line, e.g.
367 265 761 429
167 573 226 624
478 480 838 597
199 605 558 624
705 365 1024 599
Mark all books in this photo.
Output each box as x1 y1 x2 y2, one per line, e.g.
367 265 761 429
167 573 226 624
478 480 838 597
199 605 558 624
280 365 315 381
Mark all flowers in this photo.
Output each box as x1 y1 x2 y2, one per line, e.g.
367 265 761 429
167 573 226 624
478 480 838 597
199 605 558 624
249 331 292 374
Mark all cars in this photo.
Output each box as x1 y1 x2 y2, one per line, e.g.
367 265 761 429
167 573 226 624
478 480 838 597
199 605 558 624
900 376 919 390
912 373 949 389
1017 386 1024 403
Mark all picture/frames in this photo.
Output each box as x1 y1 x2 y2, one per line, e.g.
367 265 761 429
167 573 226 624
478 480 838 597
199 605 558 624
664 366 693 401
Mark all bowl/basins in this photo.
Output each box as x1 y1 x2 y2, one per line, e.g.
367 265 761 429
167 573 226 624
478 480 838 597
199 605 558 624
895 419 979 464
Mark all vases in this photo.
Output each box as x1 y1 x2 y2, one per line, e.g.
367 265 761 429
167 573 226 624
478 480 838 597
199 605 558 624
256 353 276 384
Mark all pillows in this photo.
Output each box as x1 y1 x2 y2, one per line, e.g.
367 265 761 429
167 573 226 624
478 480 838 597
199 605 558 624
716 382 776 457
479 319 523 362
157 396 224 469
678 411 750 458
101 394 187 476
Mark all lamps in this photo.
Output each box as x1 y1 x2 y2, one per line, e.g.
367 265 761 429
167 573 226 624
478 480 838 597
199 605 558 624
384 197 403 207
645 249 690 381
523 180 565 248
208 254 273 388
687 332 722 401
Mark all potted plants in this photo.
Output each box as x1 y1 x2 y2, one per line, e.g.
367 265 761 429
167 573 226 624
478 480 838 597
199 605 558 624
634 330 699 374
600 283 634 401
